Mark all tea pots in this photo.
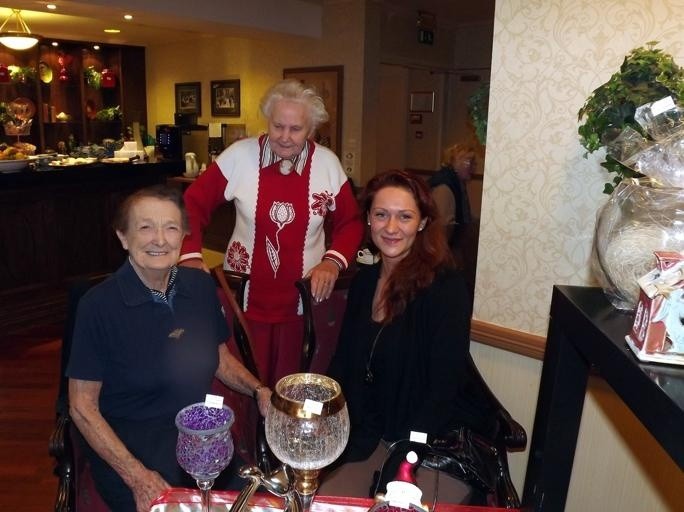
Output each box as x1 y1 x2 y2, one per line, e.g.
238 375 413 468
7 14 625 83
55 111 72 122
183 152 199 178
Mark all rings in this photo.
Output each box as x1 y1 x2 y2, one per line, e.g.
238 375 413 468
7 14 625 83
324 280 330 286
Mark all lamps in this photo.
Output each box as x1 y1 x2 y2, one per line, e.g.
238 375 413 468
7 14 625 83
0 7 43 51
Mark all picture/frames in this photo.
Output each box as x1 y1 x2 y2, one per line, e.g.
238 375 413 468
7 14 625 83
409 91 435 124
175 82 201 117
211 79 241 117
283 65 342 162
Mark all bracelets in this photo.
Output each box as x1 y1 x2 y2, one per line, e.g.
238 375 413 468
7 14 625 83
249 381 267 400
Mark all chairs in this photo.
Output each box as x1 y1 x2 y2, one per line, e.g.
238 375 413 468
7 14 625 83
49 269 272 511
295 274 526 511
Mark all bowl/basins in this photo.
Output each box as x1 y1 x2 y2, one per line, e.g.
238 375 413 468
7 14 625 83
0 160 30 172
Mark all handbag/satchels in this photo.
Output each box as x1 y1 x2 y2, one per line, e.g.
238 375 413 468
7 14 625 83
381 406 508 490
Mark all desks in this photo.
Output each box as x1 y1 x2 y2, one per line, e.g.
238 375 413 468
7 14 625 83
519 284 684 512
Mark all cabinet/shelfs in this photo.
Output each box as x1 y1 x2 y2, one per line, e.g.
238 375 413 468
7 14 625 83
0 34 146 154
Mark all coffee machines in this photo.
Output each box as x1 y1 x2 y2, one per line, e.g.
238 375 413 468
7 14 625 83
155 124 210 177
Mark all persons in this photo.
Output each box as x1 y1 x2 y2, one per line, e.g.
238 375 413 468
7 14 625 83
176 78 365 465
326 169 512 505
424 142 478 244
64 186 273 512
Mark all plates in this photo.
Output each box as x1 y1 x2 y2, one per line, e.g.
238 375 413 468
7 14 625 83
39 62 54 86
